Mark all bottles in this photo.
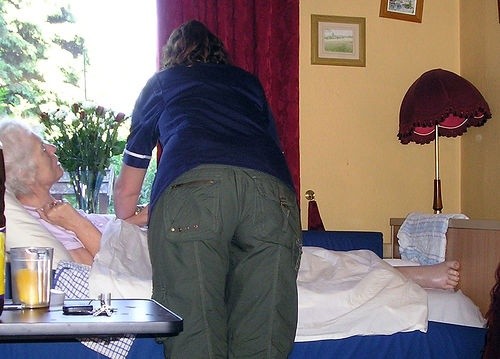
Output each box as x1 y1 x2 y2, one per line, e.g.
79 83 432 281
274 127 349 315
0 142 6 322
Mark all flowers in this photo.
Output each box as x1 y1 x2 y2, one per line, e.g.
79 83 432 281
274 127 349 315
41 103 129 166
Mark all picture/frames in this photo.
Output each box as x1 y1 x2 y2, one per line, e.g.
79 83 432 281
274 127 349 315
378 0 424 23
311 14 366 67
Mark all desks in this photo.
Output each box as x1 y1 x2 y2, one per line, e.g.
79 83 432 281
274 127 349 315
390 218 500 318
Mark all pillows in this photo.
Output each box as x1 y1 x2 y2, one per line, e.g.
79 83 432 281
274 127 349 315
4 189 74 269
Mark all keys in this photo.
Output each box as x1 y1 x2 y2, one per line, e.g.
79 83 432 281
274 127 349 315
93 305 117 316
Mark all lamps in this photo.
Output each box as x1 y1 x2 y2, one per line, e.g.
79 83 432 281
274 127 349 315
397 69 492 214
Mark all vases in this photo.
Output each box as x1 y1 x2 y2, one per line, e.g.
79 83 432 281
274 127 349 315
70 170 103 214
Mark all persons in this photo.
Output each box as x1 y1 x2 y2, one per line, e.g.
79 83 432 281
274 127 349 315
114 20 303 359
0 120 460 289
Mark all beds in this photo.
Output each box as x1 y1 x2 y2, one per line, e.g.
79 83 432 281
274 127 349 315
0 149 488 359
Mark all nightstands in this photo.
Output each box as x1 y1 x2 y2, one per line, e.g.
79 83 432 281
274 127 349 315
0 297 184 359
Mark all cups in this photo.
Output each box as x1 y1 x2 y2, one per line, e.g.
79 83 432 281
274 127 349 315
10 247 54 309
